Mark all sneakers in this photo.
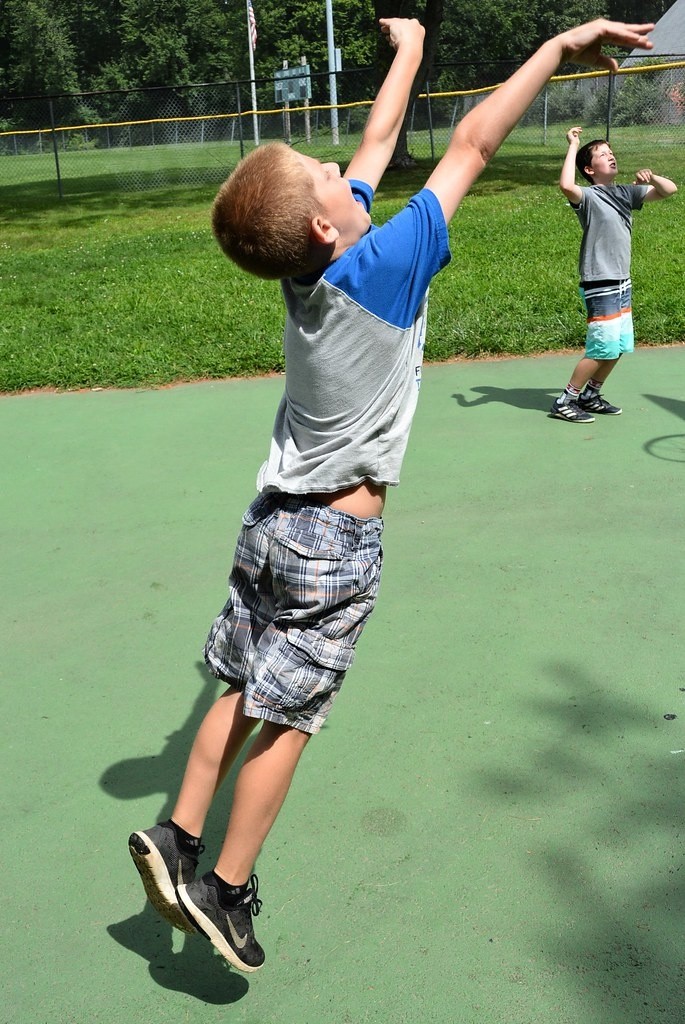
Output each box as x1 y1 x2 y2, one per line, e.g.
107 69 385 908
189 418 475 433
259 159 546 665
177 871 265 973
578 393 622 414
550 397 595 422
129 822 205 935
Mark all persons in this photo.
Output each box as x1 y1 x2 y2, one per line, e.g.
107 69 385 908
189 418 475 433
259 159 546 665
127 18 654 971
551 125 678 424
